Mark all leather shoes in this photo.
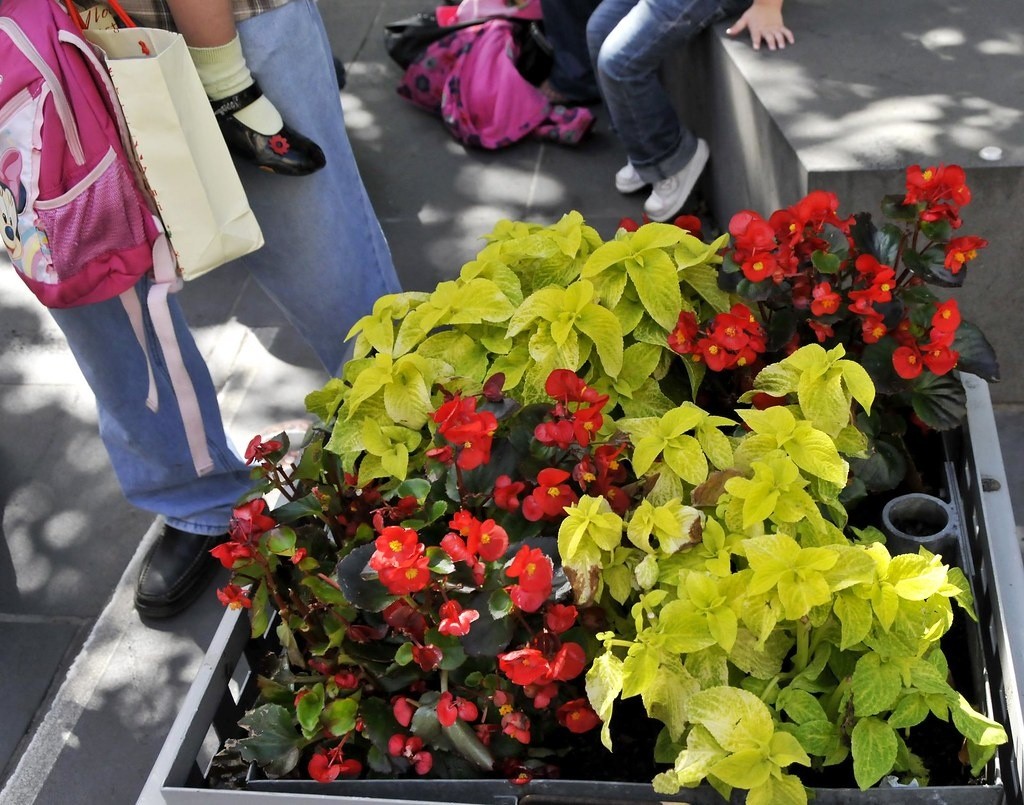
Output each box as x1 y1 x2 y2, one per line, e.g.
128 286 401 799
132 524 230 617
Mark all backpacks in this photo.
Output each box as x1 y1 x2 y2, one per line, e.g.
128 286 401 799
0 1 163 312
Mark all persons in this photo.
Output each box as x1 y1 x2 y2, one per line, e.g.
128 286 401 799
0 0 405 621
586 0 795 221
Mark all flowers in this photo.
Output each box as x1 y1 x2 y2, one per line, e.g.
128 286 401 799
207 165 1007 805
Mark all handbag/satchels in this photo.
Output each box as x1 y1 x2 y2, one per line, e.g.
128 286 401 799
380 12 448 70
66 0 267 281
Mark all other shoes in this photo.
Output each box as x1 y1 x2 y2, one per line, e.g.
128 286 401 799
639 138 710 223
614 155 644 195
538 78 602 107
206 81 330 177
